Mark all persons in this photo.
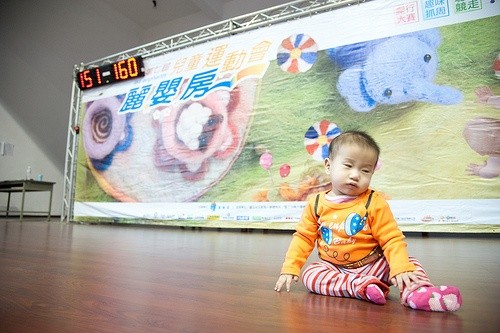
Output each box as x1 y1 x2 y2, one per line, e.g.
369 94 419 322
274 126 462 311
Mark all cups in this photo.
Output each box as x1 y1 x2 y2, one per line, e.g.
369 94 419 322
39 175 43 181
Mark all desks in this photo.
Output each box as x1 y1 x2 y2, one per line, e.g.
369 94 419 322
0 180 56 222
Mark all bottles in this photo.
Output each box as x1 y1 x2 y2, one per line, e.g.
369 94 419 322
27 166 31 180
